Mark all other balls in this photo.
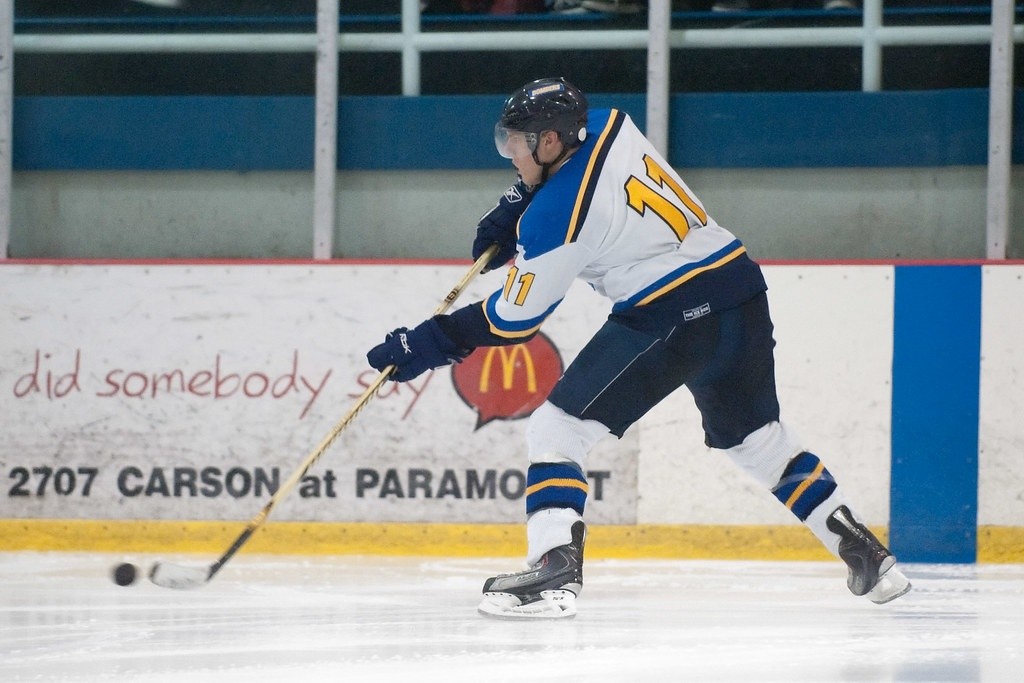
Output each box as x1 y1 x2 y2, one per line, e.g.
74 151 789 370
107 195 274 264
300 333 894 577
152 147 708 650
116 564 135 586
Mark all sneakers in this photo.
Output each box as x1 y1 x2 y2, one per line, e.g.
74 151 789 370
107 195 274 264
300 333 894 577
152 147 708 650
826 504 911 604
477 521 587 618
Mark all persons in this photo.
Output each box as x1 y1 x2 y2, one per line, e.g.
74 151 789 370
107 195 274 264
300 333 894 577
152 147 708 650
363 1 868 90
367 77 915 620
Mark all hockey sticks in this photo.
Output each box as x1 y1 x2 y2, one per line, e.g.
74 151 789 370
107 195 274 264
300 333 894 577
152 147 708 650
148 243 498 589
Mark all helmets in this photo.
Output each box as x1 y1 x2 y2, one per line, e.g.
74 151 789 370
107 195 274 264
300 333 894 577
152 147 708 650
496 76 588 144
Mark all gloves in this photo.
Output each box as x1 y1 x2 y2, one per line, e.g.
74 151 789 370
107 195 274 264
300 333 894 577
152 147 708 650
472 178 532 274
366 313 471 383
